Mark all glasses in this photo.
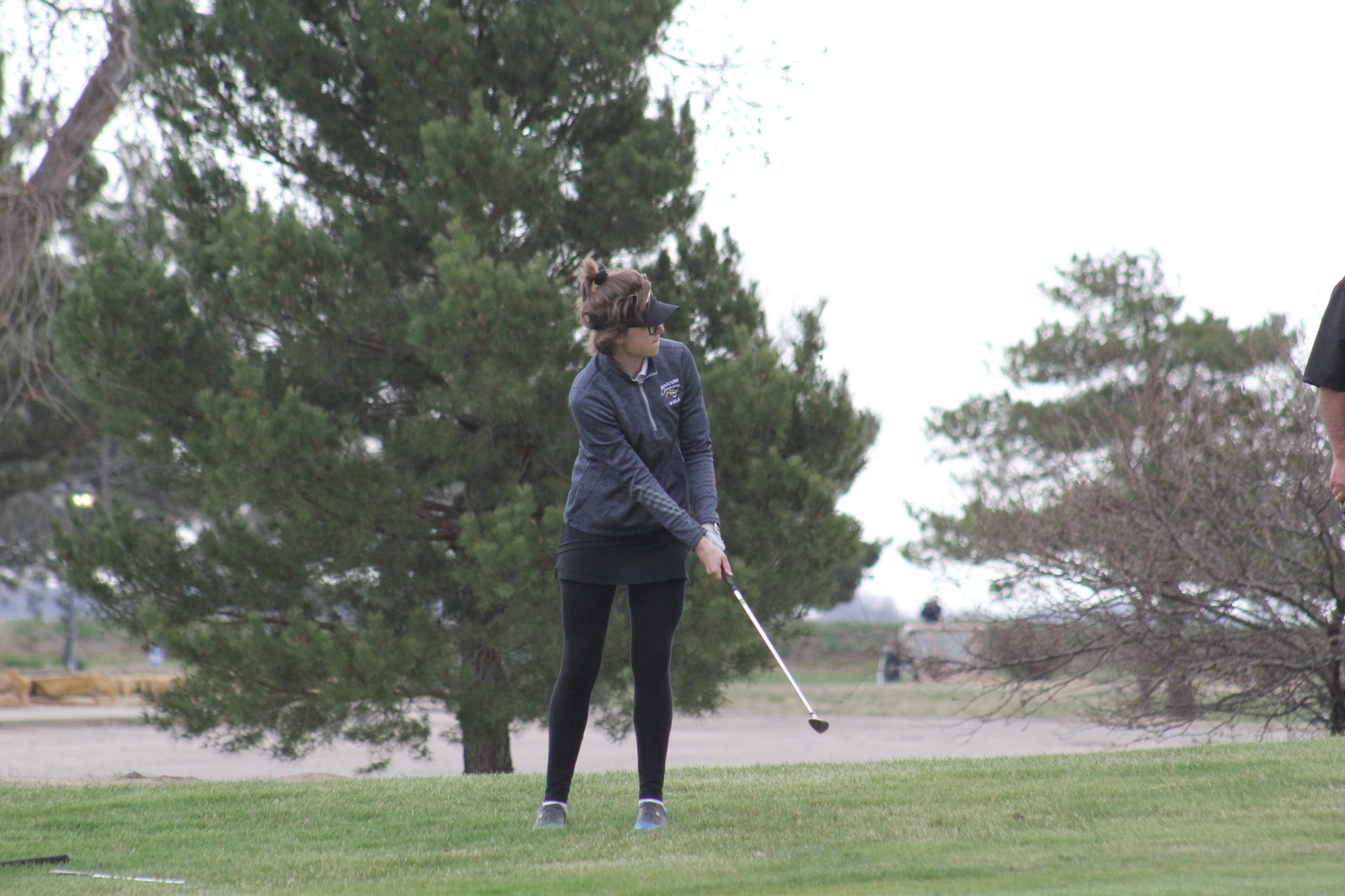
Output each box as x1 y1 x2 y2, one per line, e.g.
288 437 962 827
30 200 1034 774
648 323 664 335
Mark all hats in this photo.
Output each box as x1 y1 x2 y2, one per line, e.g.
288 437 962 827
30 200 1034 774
589 291 680 330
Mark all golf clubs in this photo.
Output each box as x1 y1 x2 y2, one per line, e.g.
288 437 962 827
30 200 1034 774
720 565 830 734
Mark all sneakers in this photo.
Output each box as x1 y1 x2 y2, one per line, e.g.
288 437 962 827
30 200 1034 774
633 802 665 830
533 803 567 828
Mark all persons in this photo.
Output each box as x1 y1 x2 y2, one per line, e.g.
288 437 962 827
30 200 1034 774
923 596 940 622
1302 276 1345 512
532 261 733 836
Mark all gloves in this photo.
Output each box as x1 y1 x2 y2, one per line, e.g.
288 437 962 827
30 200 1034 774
700 522 725 551
